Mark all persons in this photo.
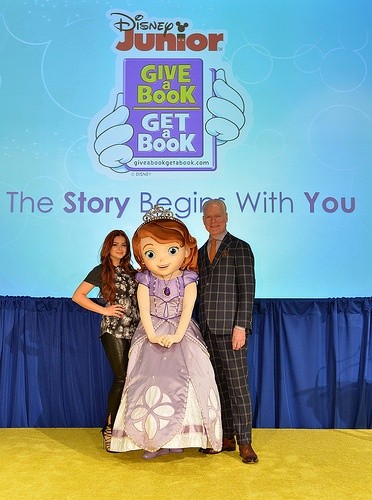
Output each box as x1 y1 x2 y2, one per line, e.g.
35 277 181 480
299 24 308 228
71 230 139 453
108 204 223 459
191 198 258 464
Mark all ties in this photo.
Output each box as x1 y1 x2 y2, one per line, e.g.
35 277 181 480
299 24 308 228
209 238 216 264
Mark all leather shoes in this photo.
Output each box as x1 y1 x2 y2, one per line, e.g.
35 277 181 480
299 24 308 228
239 444 258 462
198 437 236 455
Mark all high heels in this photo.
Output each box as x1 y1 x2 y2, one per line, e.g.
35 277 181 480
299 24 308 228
99 424 117 453
